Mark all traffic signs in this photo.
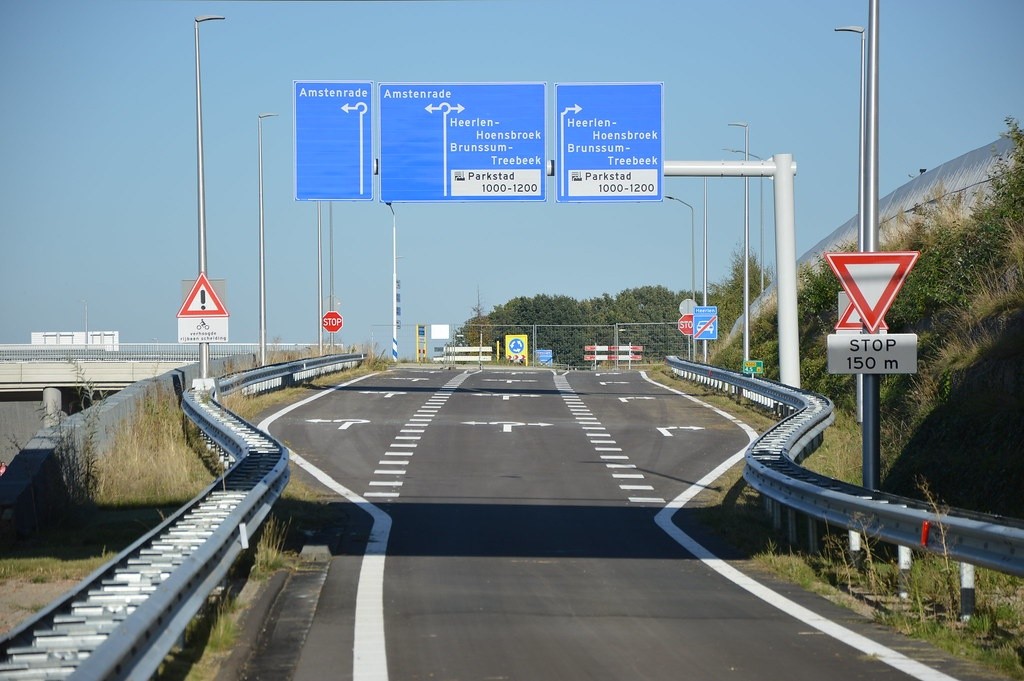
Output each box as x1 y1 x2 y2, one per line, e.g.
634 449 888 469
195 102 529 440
376 81 546 203
292 78 376 202
554 81 663 203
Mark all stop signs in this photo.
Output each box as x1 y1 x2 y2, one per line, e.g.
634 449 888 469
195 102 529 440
322 310 343 333
678 314 693 335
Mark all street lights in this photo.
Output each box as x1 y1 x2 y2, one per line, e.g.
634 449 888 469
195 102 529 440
727 121 748 374
258 111 279 366
664 195 698 362
834 24 866 424
385 202 399 363
720 147 765 296
193 14 225 380
